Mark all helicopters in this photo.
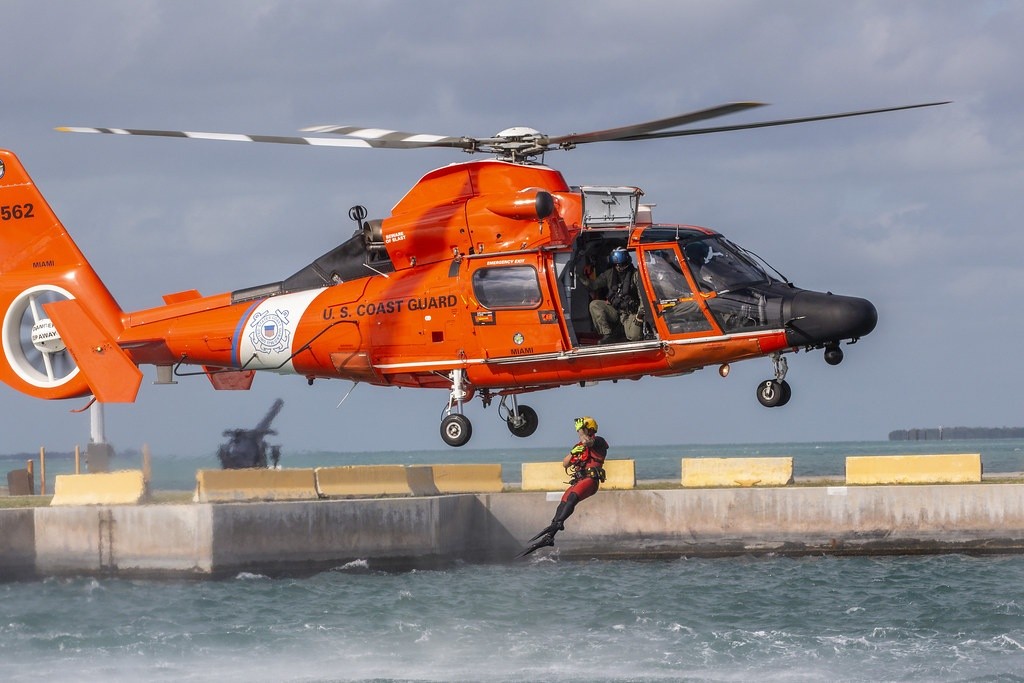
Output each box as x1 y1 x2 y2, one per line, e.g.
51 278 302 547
0 102 953 449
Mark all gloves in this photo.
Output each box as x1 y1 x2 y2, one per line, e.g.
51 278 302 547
569 270 581 279
574 418 588 432
570 445 585 455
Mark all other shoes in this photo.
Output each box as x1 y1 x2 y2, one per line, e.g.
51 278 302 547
597 334 616 344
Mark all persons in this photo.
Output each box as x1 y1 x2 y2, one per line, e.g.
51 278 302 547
576 246 644 344
650 239 708 299
514 416 609 559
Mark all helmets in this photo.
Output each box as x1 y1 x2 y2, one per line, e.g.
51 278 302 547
583 416 598 433
685 241 708 258
609 246 629 263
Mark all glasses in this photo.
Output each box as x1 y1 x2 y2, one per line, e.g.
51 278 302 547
613 264 625 268
